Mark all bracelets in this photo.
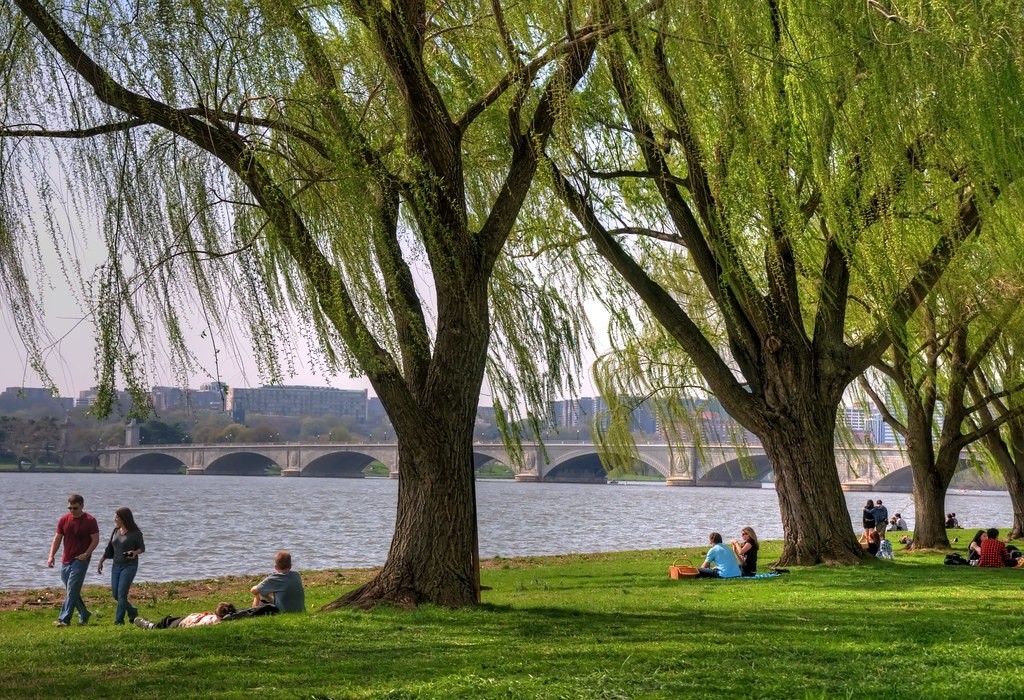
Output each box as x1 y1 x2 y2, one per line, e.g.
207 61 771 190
84 553 88 558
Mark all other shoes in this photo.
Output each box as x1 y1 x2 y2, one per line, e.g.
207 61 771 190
134 618 150 629
54 620 70 627
78 612 91 625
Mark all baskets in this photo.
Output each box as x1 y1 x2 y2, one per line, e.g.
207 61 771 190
669 558 701 580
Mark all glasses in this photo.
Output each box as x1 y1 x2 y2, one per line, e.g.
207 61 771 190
68 505 81 510
742 533 747 536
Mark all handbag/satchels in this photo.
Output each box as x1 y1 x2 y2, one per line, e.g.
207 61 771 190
104 528 116 558
944 552 963 565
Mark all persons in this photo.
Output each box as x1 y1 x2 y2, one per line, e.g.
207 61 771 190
945 513 958 529
48 495 99 627
98 507 145 625
858 531 894 559
968 530 987 565
887 513 907 531
979 528 1010 568
863 499 888 540
731 527 759 577
695 532 741 578
134 602 236 630
251 552 304 613
